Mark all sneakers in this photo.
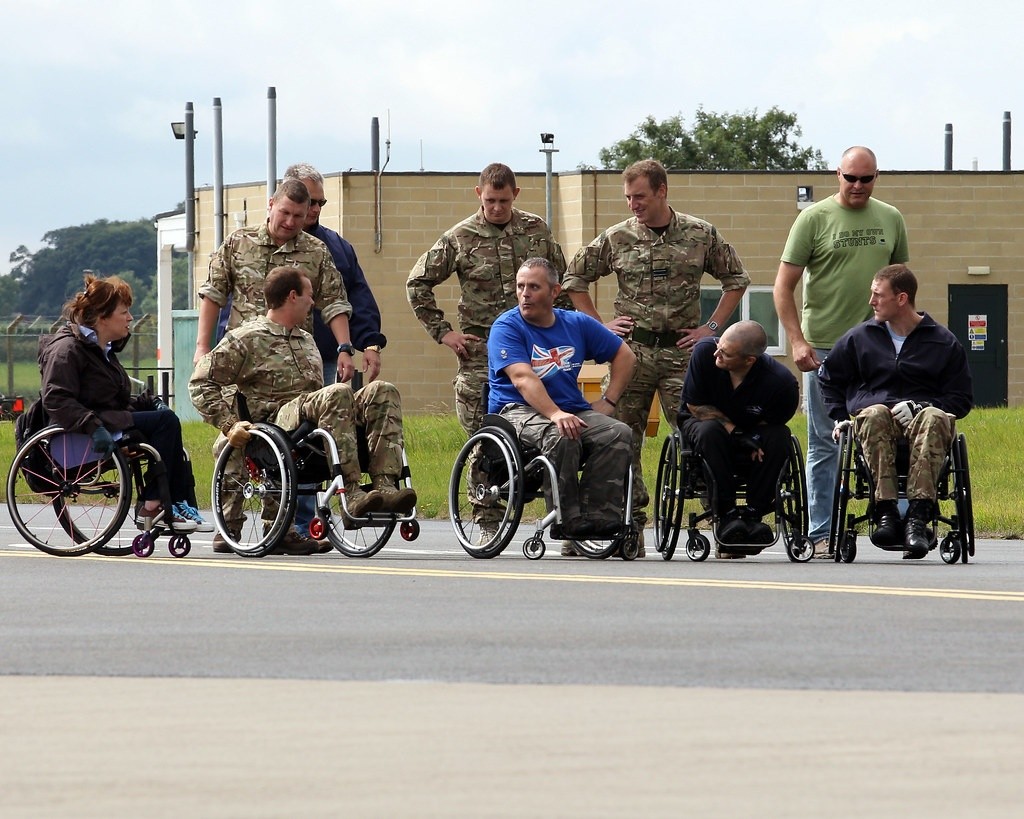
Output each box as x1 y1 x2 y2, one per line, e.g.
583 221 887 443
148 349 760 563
136 505 198 530
375 475 417 512
178 500 214 533
872 514 905 544
904 515 931 553
338 488 384 530
261 527 317 555
213 529 242 553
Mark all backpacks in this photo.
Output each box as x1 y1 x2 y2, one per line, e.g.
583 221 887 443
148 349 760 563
14 396 55 495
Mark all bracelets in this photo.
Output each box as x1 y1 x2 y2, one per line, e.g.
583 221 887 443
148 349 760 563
602 395 616 407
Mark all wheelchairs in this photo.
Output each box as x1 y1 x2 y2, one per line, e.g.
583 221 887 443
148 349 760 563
208 390 423 560
446 381 643 561
651 412 816 564
6 387 192 561
825 424 978 565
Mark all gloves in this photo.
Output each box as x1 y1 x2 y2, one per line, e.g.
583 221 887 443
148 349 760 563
92 427 114 455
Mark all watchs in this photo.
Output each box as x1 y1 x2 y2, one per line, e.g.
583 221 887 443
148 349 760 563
336 345 355 357
365 345 382 353
706 319 719 333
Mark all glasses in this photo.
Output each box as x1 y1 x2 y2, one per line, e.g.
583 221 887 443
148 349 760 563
714 339 741 360
843 174 876 184
310 198 327 206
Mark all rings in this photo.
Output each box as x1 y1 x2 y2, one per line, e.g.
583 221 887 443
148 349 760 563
690 339 696 345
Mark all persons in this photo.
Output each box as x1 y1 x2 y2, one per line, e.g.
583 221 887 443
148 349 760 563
217 162 386 551
185 264 418 518
773 145 912 560
561 159 751 556
814 265 973 561
676 319 800 555
487 256 640 528
37 272 215 532
195 179 355 557
403 162 586 556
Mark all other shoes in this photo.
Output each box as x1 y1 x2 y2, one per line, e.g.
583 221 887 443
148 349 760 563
813 539 837 560
714 543 745 559
476 524 501 550
550 517 594 540
592 517 624 540
314 538 333 554
611 530 645 558
716 518 747 553
562 538 603 556
746 522 773 555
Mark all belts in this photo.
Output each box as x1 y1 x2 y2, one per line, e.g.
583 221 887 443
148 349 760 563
465 326 491 340
624 325 682 347
